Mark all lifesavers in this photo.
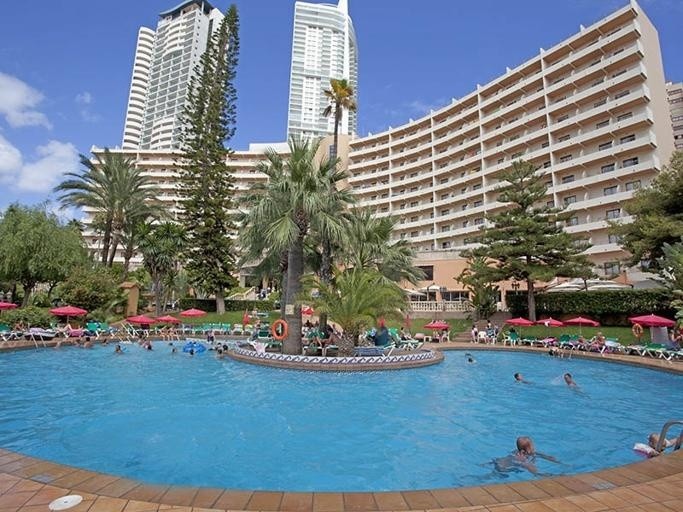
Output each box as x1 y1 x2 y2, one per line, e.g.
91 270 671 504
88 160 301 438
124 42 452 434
272 319 288 340
632 323 643 340
183 342 205 353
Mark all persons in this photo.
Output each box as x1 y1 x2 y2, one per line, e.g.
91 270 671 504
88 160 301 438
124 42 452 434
554 349 558 356
462 433 573 480
251 306 258 318
575 334 588 353
563 373 575 386
252 319 259 338
547 346 552 356
135 325 228 360
590 331 607 356
466 357 477 364
632 427 679 457
677 327 682 340
74 339 80 347
82 337 92 349
471 319 517 346
53 320 72 333
513 372 523 384
12 319 26 332
300 318 451 350
114 344 121 353
100 336 108 347
664 329 682 351
255 317 260 328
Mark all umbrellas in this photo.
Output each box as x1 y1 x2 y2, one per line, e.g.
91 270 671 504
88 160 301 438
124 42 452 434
242 308 249 327
532 316 563 338
504 316 533 341
378 313 385 327
48 305 88 324
155 313 181 327
301 308 314 316
178 307 206 324
403 314 411 329
0 301 17 312
124 314 154 330
561 315 600 336
628 312 674 342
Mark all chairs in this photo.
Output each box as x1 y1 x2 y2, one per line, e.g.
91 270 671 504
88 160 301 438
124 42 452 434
1 317 450 358
475 330 683 367
